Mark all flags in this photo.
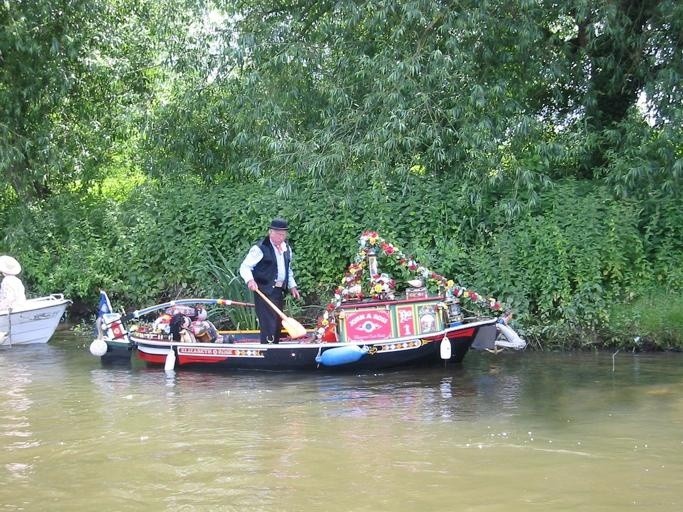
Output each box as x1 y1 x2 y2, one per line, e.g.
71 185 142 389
93 293 110 338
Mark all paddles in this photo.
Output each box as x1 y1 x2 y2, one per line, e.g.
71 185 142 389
256 289 306 339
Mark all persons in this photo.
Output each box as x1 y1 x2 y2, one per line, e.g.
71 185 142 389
496 309 512 338
237 219 300 343
0 255 27 306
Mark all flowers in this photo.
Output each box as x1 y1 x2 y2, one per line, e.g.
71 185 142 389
313 230 368 341
368 231 511 314
367 271 396 301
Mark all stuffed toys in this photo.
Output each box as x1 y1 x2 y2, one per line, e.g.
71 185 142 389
169 312 198 343
193 307 234 344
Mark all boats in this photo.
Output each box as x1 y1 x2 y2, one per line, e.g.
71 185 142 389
94 231 497 370
0 293 73 347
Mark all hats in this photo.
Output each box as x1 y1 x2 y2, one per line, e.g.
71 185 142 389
269 220 288 230
0 255 21 275
